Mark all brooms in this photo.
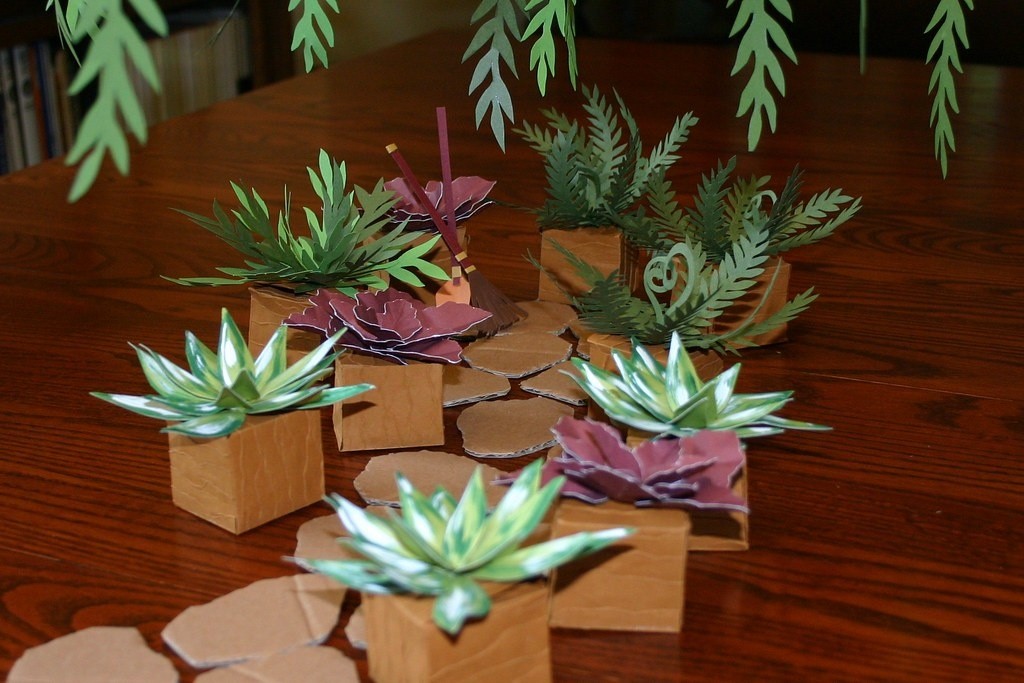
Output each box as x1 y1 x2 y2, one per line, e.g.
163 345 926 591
385 141 529 338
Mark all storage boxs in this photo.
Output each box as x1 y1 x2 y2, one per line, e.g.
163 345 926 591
362 428 752 683
364 223 472 307
167 402 326 535
332 349 445 453
669 252 793 348
585 331 727 414
538 227 639 302
246 288 341 380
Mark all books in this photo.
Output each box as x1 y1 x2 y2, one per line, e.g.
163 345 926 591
0 9 254 177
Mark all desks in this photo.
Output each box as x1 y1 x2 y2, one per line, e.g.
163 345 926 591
1 24 1024 682
